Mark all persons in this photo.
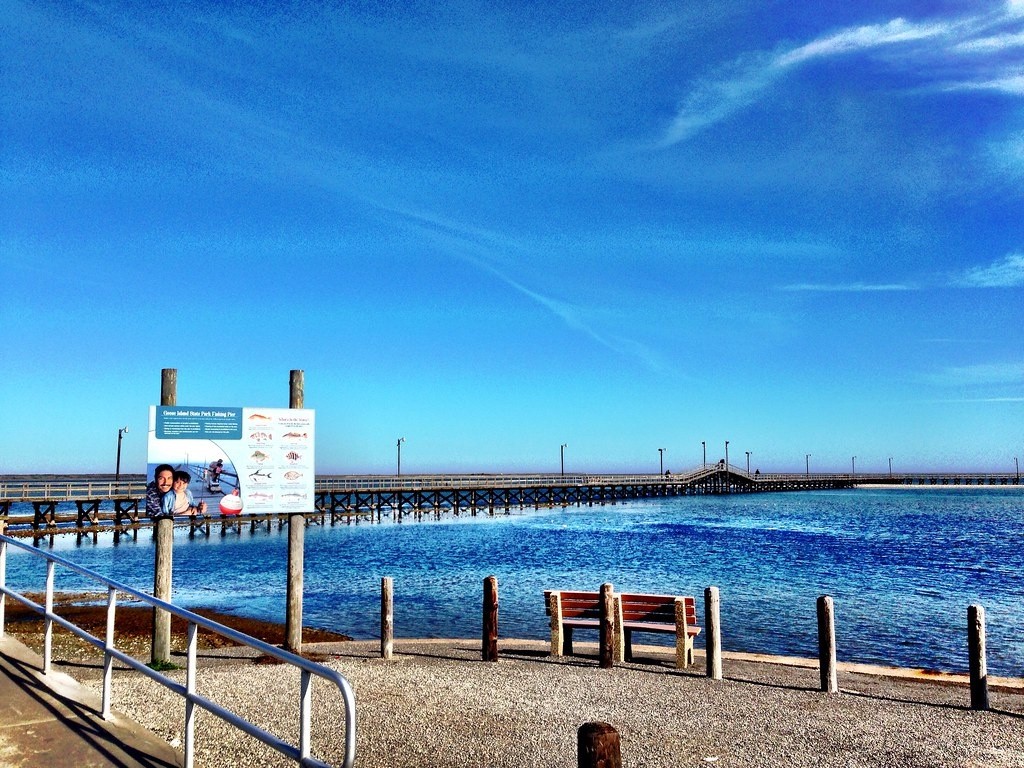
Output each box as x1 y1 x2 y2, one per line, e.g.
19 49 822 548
163 471 198 515
146 459 223 515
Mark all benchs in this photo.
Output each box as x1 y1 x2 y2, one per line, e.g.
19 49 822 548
544 590 702 665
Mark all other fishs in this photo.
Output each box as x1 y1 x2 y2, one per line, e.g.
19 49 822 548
248 414 308 502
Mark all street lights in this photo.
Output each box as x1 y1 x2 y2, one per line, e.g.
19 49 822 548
725 440 730 471
852 455 857 474
560 443 568 483
115 426 129 495
889 456 894 475
397 436 406 488
1013 457 1019 478
658 448 663 482
745 452 753 476
702 440 707 470
806 453 811 476
